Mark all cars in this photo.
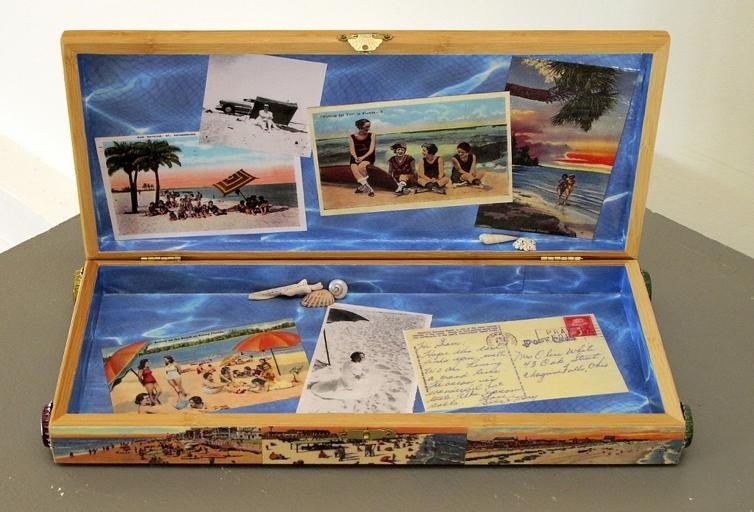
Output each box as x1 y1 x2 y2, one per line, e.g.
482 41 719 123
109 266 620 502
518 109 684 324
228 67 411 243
213 99 255 117
184 443 193 450
465 442 607 464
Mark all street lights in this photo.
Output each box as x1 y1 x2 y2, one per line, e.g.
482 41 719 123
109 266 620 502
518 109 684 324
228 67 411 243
268 425 273 437
227 427 231 445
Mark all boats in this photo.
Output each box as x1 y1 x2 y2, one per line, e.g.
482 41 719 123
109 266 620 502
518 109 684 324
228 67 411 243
464 132 507 163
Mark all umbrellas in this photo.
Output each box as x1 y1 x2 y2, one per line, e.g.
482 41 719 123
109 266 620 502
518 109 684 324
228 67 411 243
231 328 301 376
105 340 150 391
211 167 260 201
323 307 369 366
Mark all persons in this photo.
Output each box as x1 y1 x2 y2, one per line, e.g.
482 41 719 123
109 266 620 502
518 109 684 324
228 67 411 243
556 173 568 205
161 356 188 400
188 396 205 410
139 183 269 221
310 351 385 399
253 102 280 132
134 393 159 415
563 174 575 205
347 118 486 196
195 354 298 394
